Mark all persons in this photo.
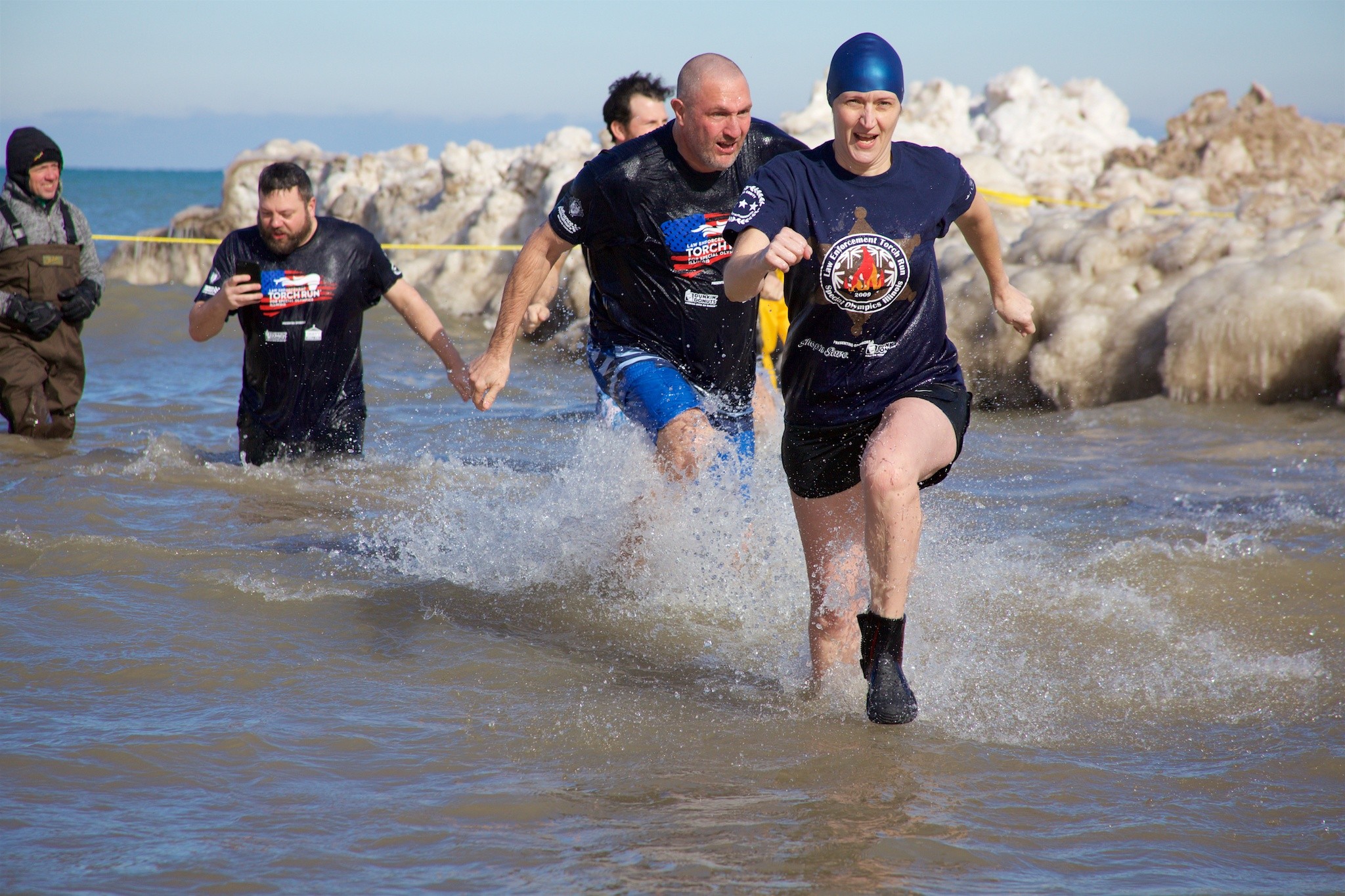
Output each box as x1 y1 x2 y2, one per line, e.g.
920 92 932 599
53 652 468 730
723 33 1035 724
0 128 106 437
467 51 814 474
523 70 782 419
190 163 474 468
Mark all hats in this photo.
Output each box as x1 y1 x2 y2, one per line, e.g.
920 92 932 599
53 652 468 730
29 148 60 168
826 32 904 108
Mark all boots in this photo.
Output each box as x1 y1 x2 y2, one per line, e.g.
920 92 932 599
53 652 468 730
856 608 918 725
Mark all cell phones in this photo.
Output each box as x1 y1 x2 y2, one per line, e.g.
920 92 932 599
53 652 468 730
236 259 262 310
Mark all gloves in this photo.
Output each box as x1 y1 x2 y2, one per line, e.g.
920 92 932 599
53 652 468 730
3 292 64 342
57 278 101 327
